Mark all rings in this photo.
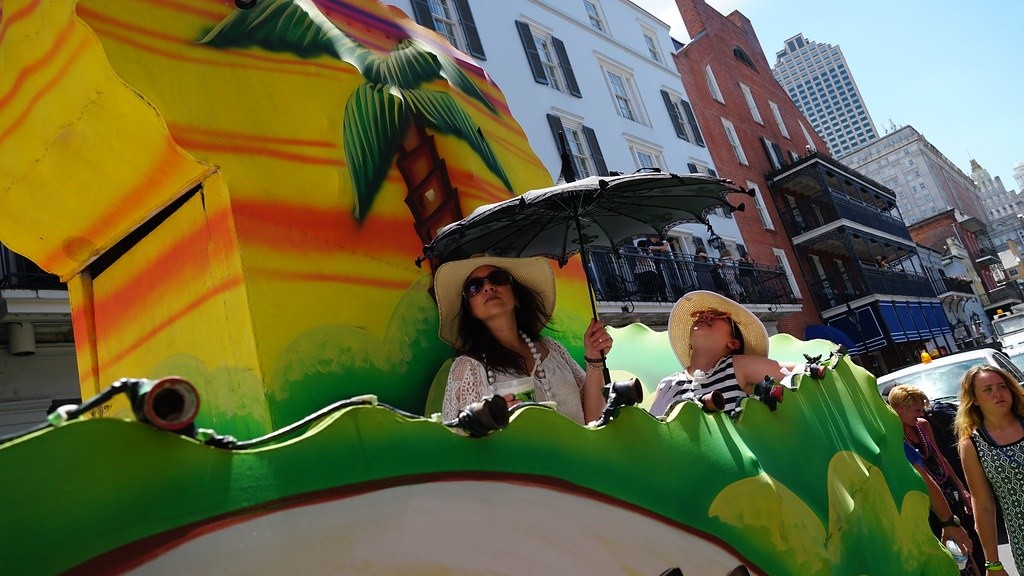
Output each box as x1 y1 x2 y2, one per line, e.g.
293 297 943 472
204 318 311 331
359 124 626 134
599 339 602 342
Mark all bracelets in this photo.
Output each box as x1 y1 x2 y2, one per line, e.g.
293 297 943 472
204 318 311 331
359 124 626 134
584 354 604 370
586 357 607 363
985 563 1003 570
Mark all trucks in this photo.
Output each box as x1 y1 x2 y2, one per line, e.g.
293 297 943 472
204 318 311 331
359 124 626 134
992 305 1024 347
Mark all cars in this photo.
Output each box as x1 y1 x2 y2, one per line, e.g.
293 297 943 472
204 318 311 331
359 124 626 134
877 346 1024 407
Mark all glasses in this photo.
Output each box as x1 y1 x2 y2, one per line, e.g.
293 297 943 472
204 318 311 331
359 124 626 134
462 269 512 299
689 310 734 334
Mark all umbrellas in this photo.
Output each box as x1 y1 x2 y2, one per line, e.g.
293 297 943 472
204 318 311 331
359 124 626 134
423 169 751 382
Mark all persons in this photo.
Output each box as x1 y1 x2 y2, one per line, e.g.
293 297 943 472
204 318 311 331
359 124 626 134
693 246 760 303
650 290 826 422
634 236 668 301
435 253 613 432
880 365 1024 576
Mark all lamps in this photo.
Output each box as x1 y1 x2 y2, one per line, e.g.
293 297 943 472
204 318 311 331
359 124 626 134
10 322 35 355
706 219 723 249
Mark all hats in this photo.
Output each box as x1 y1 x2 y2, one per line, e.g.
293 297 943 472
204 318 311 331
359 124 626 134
668 290 769 376
434 252 557 351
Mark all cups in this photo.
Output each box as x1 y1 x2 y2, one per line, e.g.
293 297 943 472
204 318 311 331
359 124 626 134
945 540 968 570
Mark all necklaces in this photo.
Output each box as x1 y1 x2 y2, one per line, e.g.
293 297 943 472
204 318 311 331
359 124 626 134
483 332 558 411
903 424 948 484
684 357 725 380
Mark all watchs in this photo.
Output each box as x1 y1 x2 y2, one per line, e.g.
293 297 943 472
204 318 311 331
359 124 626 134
942 515 961 528
